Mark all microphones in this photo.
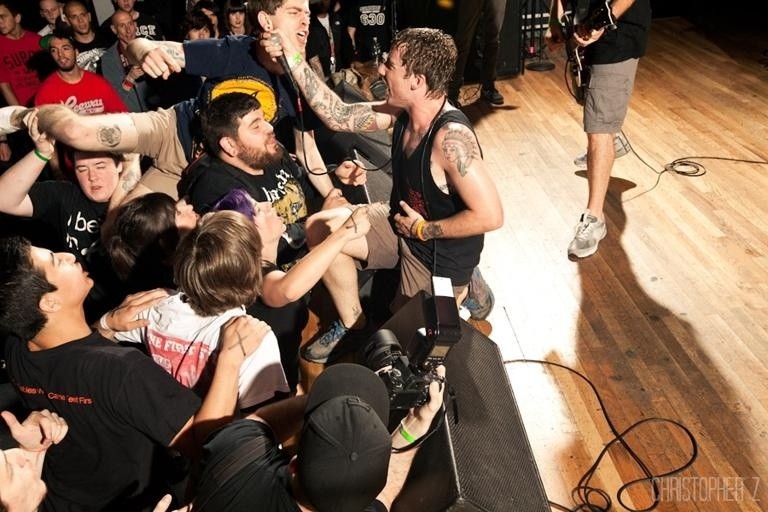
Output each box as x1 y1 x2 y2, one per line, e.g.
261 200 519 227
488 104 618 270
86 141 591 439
265 33 301 95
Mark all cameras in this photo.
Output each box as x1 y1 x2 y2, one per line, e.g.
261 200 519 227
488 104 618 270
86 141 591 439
356 276 463 418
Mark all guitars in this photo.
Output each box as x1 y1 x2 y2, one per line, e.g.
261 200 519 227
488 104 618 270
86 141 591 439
564 1 618 90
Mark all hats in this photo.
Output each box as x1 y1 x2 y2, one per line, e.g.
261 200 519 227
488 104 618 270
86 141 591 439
293 363 393 512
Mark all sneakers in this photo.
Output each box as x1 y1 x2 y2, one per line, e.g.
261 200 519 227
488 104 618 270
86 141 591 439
567 209 609 257
479 84 503 105
575 133 631 166
304 320 354 366
462 267 495 320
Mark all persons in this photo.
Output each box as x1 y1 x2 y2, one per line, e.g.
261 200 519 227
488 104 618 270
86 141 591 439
1 1 513 512
544 0 649 258
